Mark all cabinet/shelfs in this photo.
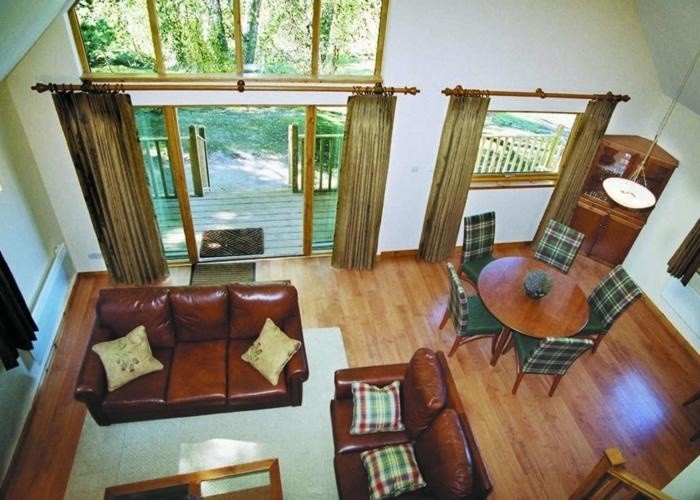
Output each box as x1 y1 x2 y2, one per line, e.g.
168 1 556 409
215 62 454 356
566 135 678 267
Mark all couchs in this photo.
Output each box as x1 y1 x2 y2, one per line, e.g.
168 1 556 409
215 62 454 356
73 283 309 426
330 352 493 500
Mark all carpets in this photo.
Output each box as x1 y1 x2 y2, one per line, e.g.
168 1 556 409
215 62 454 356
200 228 265 257
63 327 350 500
188 262 256 285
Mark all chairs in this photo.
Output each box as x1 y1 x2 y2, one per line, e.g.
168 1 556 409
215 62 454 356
440 212 642 397
683 389 700 441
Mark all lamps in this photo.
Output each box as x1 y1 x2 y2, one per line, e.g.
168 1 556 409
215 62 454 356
601 48 700 209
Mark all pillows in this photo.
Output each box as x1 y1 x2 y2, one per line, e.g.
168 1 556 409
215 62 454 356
359 436 428 500
403 347 447 440
349 379 406 435
167 285 230 342
92 325 166 393
414 405 477 500
226 279 297 340
240 317 302 386
96 287 177 348
